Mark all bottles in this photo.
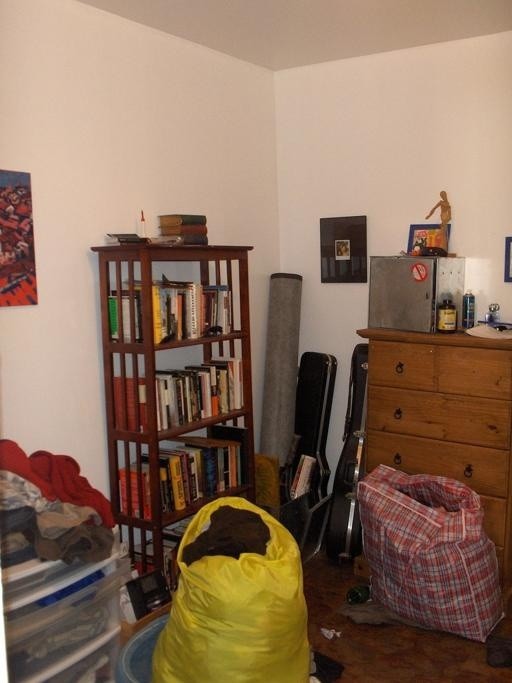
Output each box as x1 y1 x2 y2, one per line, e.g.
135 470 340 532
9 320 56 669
346 586 370 605
485 304 500 327
436 294 457 333
461 286 476 329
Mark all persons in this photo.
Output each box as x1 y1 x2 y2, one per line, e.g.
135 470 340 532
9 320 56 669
426 189 451 255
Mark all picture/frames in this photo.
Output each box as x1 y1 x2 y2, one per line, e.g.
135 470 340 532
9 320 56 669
320 216 368 283
505 237 512 282
407 224 450 257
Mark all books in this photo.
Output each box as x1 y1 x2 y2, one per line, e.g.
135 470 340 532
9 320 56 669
131 514 198 590
155 213 209 248
115 423 249 520
110 357 243 433
105 276 230 344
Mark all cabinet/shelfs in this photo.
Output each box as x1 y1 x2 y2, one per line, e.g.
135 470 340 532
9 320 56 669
91 245 255 586
355 329 511 617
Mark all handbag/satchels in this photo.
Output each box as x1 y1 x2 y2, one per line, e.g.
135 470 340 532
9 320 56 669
357 462 506 642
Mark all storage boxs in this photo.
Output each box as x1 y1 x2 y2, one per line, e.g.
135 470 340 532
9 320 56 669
14 626 121 682
2 549 130 681
